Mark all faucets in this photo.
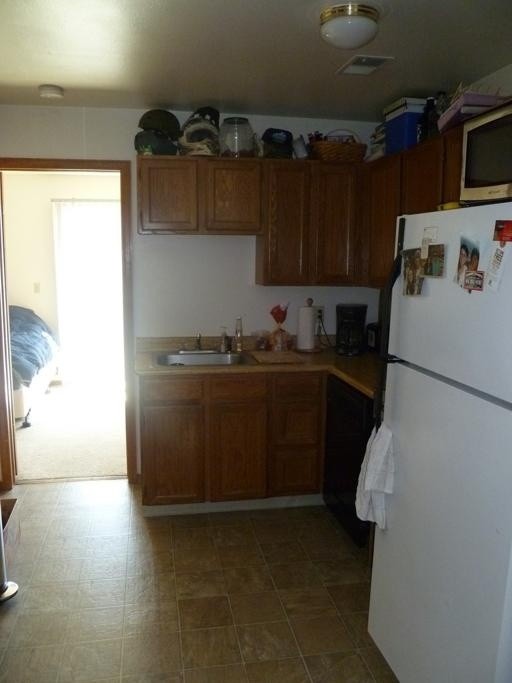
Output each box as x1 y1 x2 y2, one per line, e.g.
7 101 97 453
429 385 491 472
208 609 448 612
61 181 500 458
195 333 202 351
234 315 242 352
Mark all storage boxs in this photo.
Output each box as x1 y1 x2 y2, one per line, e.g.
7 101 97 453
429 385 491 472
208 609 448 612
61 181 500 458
2 496 22 571
382 94 424 154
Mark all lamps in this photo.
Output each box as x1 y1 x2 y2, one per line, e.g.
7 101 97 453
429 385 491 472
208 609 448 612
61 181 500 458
317 4 385 48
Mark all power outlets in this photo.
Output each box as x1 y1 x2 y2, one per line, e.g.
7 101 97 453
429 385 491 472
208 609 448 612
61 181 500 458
316 305 325 323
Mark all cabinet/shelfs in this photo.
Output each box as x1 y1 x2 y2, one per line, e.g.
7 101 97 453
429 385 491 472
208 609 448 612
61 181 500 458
366 127 467 287
135 365 268 517
254 157 365 287
268 364 327 508
136 154 263 237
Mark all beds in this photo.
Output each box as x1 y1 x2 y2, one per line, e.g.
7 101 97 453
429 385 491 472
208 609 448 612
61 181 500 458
7 301 60 428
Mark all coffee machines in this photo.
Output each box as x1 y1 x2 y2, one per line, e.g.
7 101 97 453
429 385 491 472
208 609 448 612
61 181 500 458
335 304 367 357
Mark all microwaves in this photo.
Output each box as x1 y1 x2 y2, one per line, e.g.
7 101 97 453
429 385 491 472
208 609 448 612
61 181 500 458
458 102 512 204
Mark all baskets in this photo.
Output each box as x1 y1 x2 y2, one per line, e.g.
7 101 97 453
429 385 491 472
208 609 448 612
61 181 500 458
311 128 369 162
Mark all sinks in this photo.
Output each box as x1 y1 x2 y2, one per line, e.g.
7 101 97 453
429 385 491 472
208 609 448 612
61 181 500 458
151 350 259 367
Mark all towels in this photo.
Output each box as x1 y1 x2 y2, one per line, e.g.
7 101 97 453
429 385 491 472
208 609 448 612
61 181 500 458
351 420 395 531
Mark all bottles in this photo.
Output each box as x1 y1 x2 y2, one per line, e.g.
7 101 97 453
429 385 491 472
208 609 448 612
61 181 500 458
232 316 244 352
218 116 255 158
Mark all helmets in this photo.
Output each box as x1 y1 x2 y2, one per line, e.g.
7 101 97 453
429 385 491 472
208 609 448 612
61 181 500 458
178 118 219 156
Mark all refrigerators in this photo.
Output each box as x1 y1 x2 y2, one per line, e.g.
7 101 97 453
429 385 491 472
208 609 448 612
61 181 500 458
363 200 511 681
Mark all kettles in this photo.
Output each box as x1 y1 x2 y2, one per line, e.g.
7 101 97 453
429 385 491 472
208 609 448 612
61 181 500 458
297 306 320 350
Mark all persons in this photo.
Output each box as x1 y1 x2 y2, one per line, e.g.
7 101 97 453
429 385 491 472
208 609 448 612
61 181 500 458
403 243 483 296
